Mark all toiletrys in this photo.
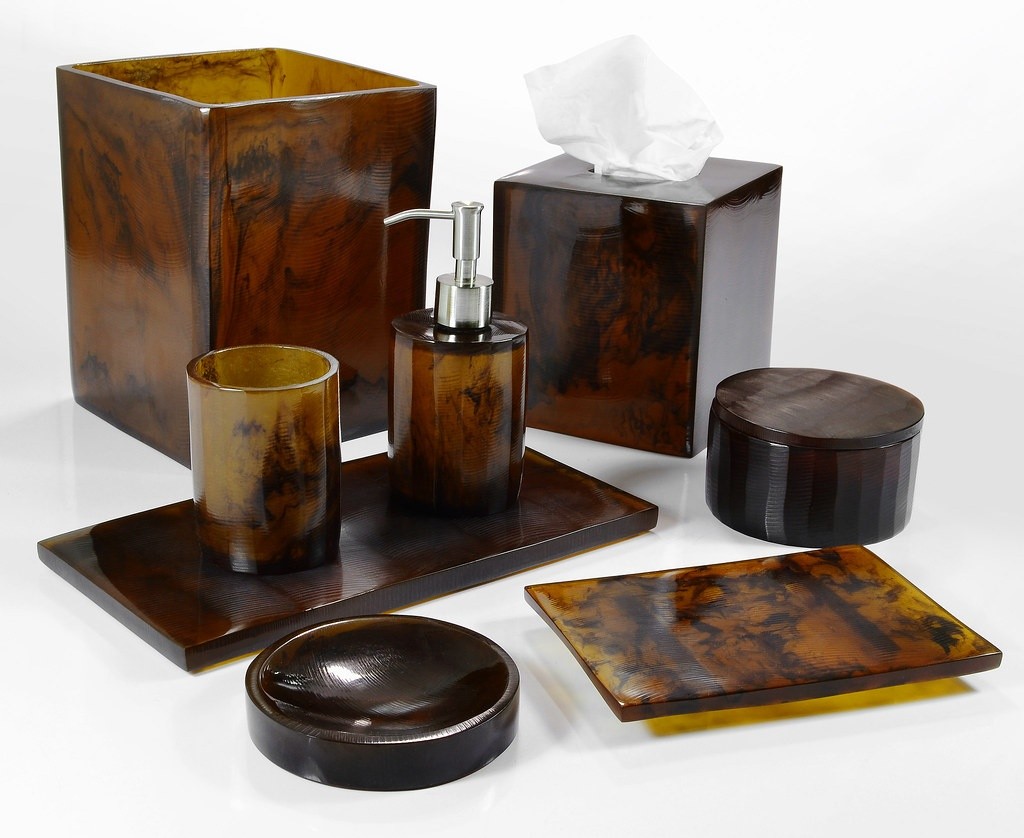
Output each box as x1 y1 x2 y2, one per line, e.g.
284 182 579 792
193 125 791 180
383 200 528 509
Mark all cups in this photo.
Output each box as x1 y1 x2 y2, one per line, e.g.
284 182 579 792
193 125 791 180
186 345 341 573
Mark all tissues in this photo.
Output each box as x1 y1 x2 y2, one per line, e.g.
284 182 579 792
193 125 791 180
495 35 783 458
